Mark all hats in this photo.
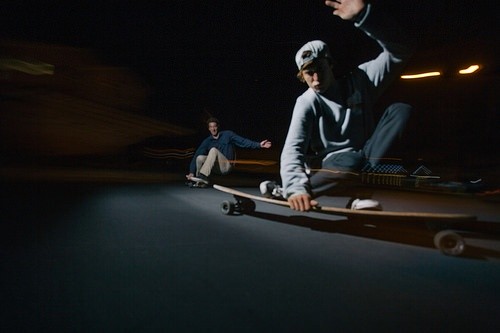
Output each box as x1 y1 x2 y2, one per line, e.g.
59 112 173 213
295 40 332 71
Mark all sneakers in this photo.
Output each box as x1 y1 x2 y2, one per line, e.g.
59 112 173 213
346 199 382 211
260 180 287 201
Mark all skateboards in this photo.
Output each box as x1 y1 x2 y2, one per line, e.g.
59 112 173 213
185 175 208 188
213 184 478 257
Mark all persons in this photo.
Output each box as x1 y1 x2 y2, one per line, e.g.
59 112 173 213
187 118 271 180
260 0 414 212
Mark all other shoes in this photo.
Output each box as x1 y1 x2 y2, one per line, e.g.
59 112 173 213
198 173 206 180
